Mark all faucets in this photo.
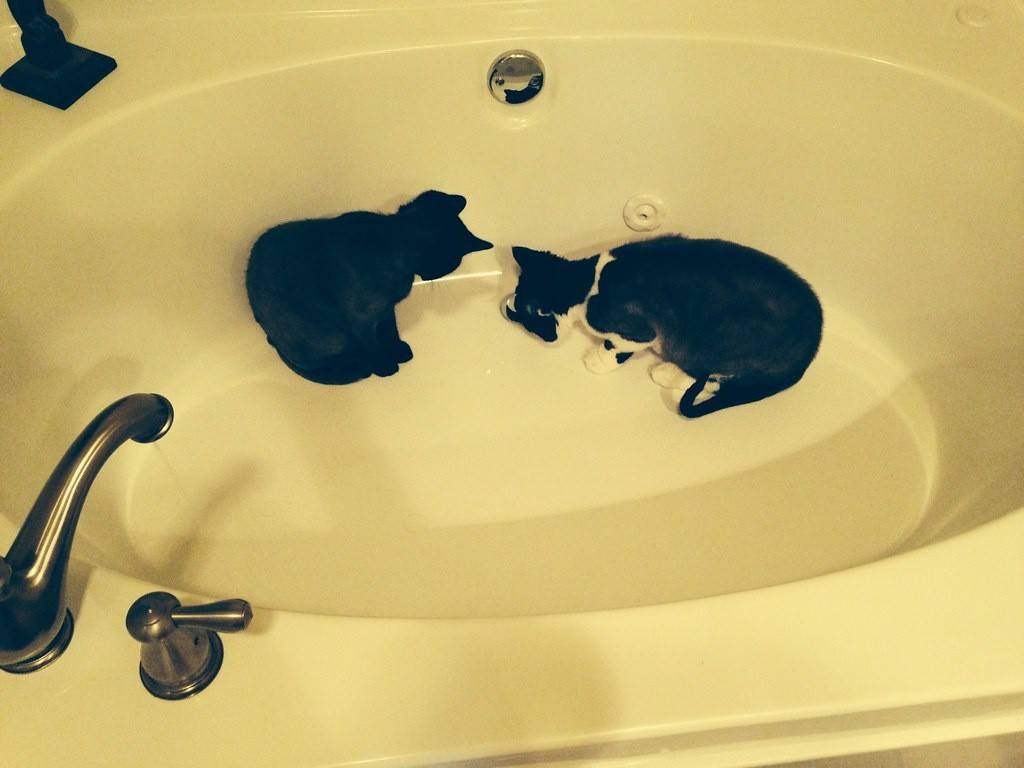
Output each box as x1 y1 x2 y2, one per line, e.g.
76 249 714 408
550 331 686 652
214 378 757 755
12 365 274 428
0 389 177 674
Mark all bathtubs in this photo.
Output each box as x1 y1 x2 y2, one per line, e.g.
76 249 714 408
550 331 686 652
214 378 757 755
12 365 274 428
0 0 1023 767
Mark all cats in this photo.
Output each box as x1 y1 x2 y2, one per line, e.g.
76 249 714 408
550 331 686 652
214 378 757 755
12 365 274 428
244 189 494 386
512 232 823 418
